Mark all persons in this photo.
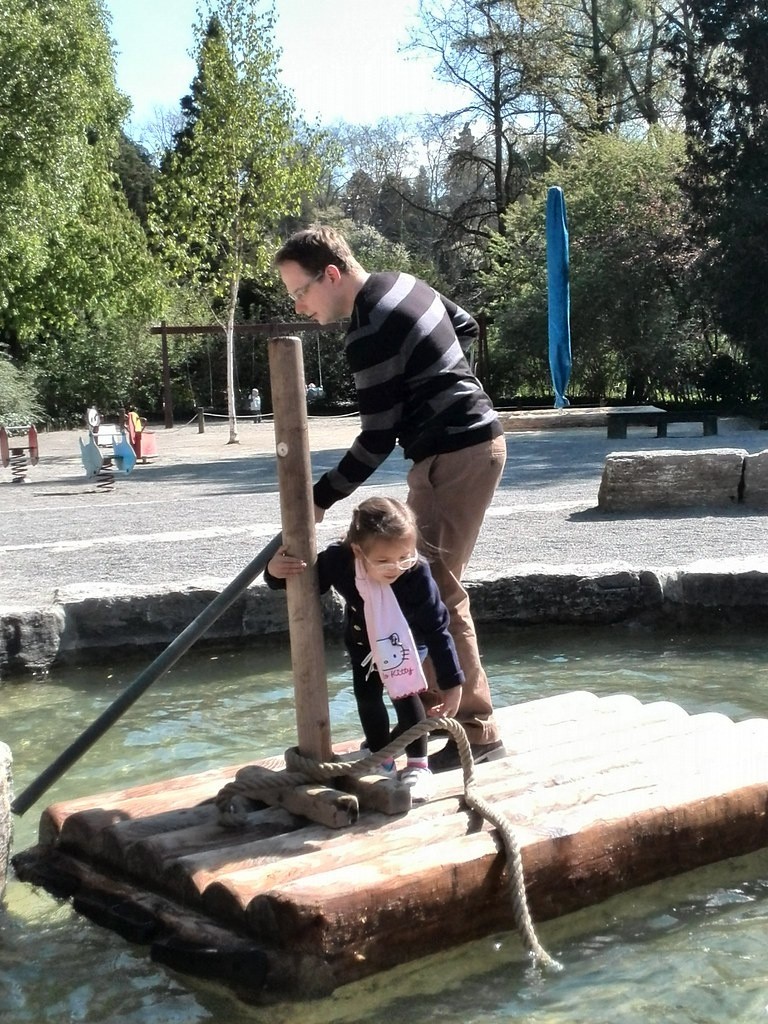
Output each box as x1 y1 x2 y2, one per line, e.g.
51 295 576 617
275 226 507 773
264 497 466 803
248 388 261 424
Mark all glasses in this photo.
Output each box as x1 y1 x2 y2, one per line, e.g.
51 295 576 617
288 269 323 302
351 542 419 573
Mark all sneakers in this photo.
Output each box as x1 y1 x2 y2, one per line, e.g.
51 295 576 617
369 758 398 781
400 766 436 802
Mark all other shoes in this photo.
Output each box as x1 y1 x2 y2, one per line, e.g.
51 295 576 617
428 739 507 774
